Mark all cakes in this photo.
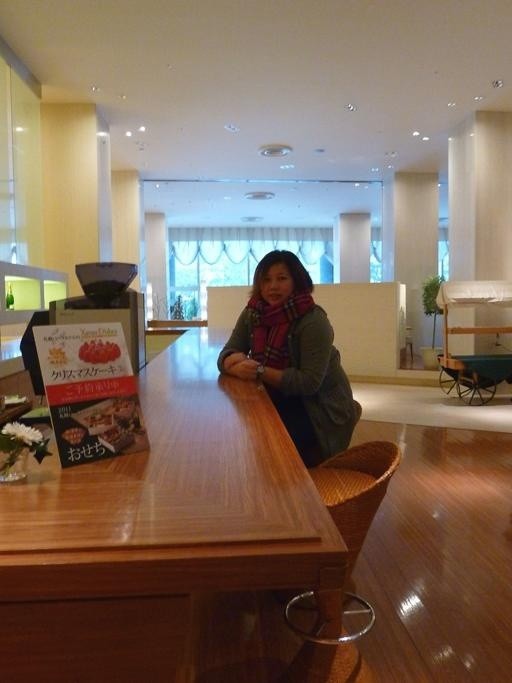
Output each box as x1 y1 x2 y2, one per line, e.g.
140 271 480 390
77 338 121 365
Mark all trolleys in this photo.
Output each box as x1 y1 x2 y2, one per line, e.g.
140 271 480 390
435 353 512 406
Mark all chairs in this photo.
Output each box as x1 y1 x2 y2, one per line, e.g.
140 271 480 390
283 440 401 646
352 399 362 431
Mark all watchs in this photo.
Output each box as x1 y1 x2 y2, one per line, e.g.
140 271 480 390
256 360 265 379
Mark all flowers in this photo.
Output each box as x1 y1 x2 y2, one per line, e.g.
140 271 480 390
0 420 52 476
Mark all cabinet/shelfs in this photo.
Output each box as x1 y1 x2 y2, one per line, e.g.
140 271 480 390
0 260 69 378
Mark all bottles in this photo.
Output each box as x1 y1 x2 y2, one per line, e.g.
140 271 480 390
7 284 15 310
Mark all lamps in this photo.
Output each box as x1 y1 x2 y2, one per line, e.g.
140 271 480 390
245 192 275 202
260 146 292 158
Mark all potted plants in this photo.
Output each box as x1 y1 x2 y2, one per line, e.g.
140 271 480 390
420 275 444 370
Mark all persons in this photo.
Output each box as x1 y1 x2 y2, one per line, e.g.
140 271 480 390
217 249 362 466
